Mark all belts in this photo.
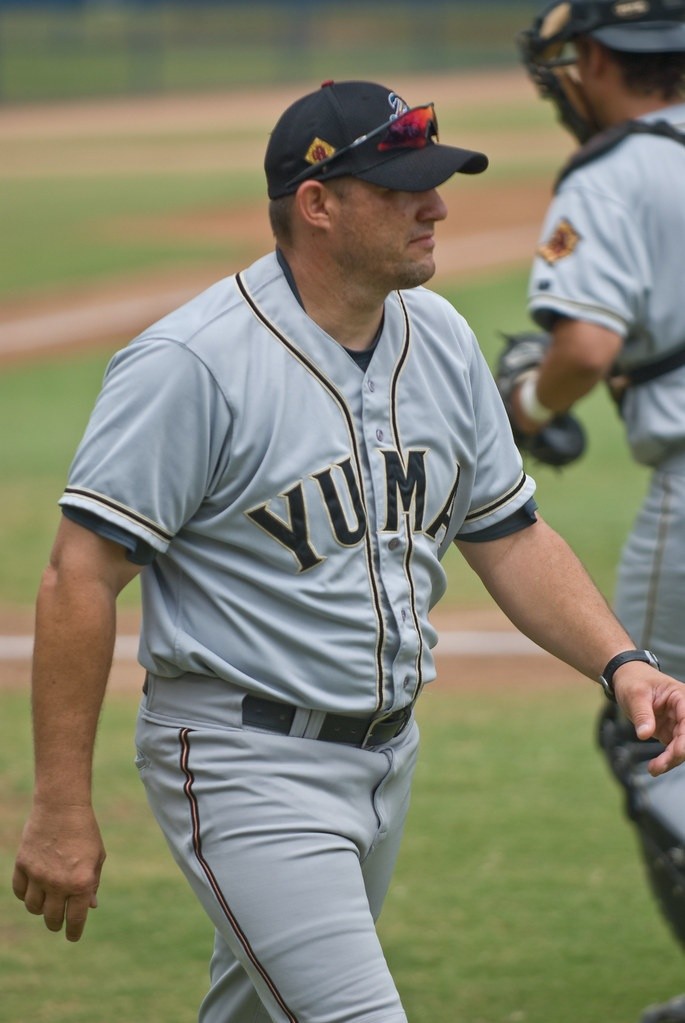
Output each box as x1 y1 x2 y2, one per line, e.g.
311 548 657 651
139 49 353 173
141 669 414 750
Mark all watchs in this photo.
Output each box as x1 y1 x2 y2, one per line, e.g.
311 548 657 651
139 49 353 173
599 649 662 703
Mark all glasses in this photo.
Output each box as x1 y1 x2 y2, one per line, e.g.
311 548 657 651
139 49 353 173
287 103 439 187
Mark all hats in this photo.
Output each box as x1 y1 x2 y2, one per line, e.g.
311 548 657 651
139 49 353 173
263 77 490 200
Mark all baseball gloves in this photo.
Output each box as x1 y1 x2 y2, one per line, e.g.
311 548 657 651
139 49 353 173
493 333 584 464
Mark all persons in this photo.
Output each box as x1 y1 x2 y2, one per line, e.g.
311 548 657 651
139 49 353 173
495 0 685 1021
13 79 684 1022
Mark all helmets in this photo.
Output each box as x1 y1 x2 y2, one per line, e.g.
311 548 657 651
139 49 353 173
575 0 685 50
